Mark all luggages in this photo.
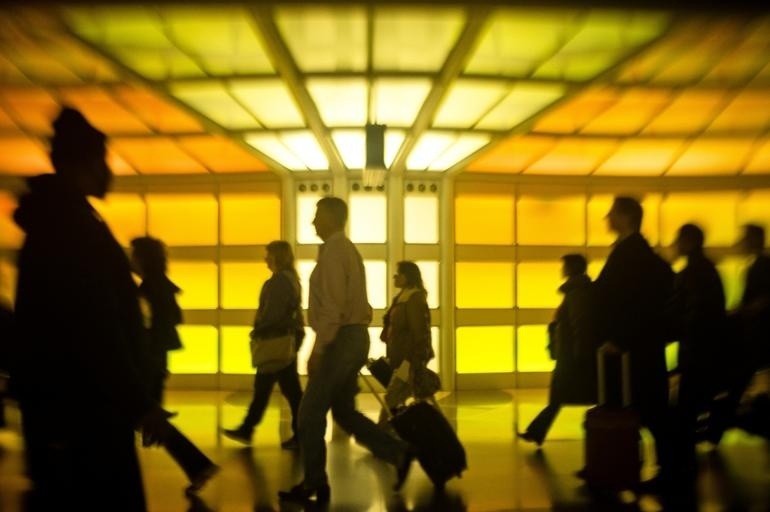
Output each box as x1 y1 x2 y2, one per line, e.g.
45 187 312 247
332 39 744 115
582 344 644 501
357 369 468 495
365 355 394 391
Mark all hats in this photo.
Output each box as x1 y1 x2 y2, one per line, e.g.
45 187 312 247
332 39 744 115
47 103 109 172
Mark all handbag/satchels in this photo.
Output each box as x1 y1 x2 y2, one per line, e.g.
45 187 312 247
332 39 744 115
250 332 298 375
409 363 441 398
548 318 559 361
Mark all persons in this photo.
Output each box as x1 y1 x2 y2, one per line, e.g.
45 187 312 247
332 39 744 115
223 241 305 449
278 198 415 506
10 105 177 512
128 236 220 497
1 301 15 459
379 260 434 430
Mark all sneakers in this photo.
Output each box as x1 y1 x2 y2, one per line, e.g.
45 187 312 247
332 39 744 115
183 464 221 495
281 436 302 451
220 425 253 447
391 440 418 493
278 480 331 504
516 428 544 447
